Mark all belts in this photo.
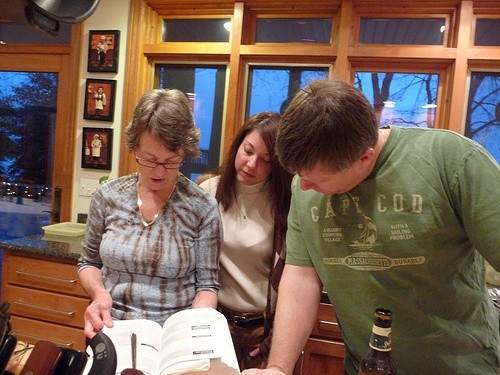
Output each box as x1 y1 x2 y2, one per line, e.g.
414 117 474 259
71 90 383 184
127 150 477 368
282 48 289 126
218 309 265 328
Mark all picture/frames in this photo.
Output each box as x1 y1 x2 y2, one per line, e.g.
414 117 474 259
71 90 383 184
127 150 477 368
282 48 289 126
82 126 114 169
83 78 117 122
87 29 120 72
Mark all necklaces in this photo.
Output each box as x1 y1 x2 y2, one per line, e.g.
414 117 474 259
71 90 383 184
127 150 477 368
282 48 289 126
132 173 176 227
235 190 262 220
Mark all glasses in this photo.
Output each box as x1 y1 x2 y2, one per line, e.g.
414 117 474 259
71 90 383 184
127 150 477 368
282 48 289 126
134 147 185 168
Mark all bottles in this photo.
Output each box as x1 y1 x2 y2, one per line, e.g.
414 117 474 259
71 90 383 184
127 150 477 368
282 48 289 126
357 307 397 375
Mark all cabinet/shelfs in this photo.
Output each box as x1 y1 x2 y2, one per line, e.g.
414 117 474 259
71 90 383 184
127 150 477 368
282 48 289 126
1 246 94 354
291 299 349 375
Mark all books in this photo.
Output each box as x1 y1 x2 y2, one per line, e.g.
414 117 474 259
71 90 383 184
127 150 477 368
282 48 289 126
81 307 240 375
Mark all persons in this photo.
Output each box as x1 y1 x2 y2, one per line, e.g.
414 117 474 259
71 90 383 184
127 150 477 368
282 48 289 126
76 87 224 341
241 80 500 375
92 86 107 115
194 111 296 372
91 134 103 162
96 35 108 65
